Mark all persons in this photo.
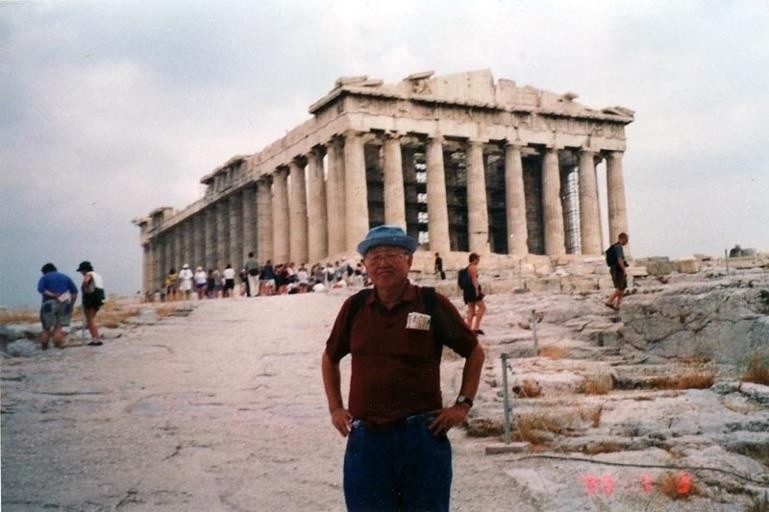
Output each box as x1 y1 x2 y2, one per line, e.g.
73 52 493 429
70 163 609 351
135 251 373 301
604 232 630 312
76 259 107 347
461 252 487 335
36 262 78 350
317 225 488 511
432 251 443 282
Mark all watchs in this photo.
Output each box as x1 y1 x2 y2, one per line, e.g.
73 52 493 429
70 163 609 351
456 394 473 407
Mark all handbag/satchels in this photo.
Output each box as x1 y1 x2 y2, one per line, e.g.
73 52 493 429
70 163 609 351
459 270 468 289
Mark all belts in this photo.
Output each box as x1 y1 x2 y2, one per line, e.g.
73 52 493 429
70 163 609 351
353 413 425 430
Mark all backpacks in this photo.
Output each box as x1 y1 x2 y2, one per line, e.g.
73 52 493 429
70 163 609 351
607 242 619 266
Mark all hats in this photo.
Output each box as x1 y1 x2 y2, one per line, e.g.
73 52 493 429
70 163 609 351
358 225 416 254
76 262 92 271
